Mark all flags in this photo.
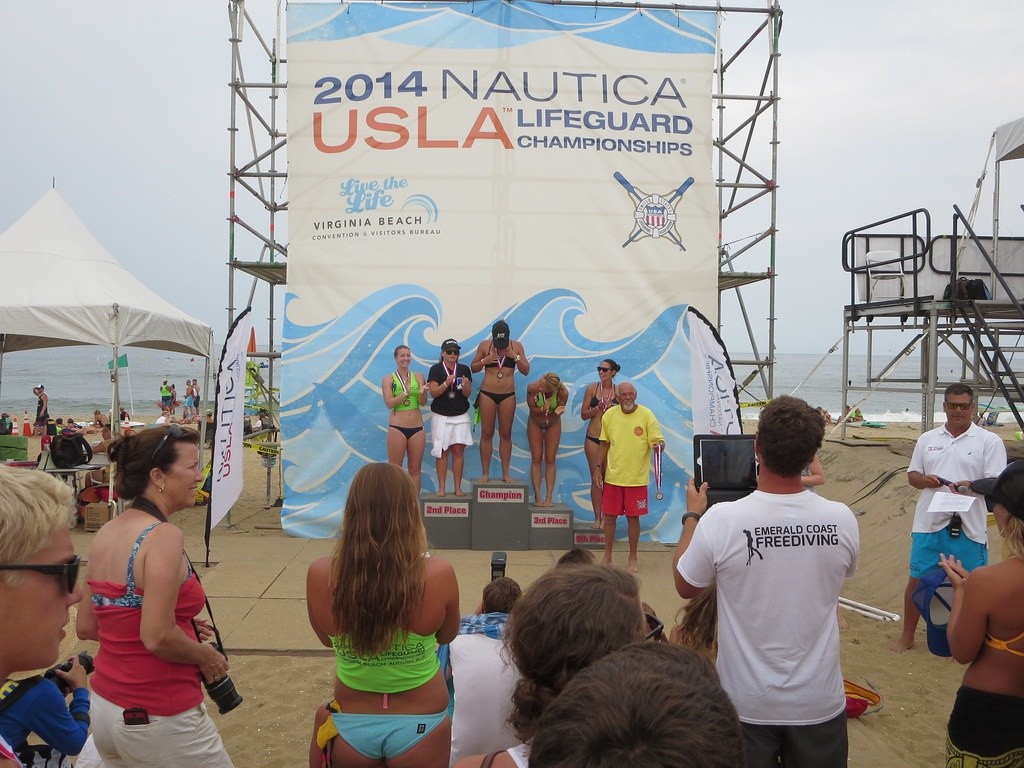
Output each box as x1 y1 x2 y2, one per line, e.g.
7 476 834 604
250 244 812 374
108 355 128 370
205 309 253 530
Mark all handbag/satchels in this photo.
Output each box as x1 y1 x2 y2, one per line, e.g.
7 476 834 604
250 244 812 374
943 277 992 300
51 433 93 469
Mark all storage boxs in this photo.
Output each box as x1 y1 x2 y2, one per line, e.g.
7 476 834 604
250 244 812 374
85 503 109 532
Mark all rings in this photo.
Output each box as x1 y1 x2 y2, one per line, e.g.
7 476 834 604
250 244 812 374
215 674 221 679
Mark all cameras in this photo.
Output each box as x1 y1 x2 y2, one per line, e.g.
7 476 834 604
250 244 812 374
490 552 507 582
204 642 243 715
45 649 96 698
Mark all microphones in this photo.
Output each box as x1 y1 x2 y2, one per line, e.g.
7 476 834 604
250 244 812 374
937 478 967 493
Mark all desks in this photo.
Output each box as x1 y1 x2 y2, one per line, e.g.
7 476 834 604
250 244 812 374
46 465 100 488
6 460 38 467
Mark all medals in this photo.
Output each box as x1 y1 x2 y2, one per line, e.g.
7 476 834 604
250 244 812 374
448 393 455 398
546 419 548 424
656 492 663 500
404 400 410 406
497 372 503 378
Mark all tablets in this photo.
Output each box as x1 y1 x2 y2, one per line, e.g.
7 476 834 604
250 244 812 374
692 434 761 493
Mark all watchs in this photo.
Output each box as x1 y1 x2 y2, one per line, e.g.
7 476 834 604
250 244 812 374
514 355 521 362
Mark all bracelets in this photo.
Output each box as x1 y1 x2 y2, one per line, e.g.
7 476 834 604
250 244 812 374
481 359 485 366
682 512 701 525
444 381 449 387
404 391 408 395
596 464 601 468
597 405 599 410
541 406 544 413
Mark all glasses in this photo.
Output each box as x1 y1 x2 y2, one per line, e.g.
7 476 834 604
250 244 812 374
946 401 973 410
152 424 182 458
643 612 664 644
597 367 613 372
444 350 459 355
0 555 81 594
186 382 189 383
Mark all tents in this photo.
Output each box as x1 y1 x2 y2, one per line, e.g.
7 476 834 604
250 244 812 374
0 187 208 413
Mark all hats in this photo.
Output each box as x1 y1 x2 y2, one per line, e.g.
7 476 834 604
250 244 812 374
110 409 112 412
492 321 510 349
163 379 168 383
35 384 44 389
257 409 268 413
912 565 953 657
206 409 212 414
441 339 461 352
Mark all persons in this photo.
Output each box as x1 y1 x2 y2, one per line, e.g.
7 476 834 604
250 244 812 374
838 406 864 425
977 410 987 427
890 383 1007 653
0 425 235 768
381 319 665 572
306 397 860 768
0 377 272 488
938 459 1024 768
812 406 833 425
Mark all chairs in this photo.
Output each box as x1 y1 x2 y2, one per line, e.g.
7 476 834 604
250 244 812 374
0 434 28 461
199 421 215 447
866 250 904 303
985 412 1000 425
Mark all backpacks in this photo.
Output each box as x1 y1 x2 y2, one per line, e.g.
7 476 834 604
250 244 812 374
190 385 197 398
